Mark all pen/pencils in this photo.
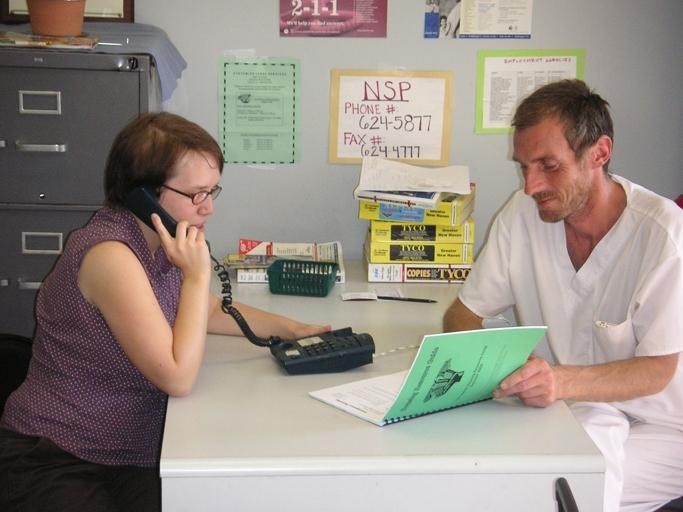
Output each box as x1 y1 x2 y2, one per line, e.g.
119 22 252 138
377 296 438 303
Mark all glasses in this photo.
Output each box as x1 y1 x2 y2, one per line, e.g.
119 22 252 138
159 183 221 205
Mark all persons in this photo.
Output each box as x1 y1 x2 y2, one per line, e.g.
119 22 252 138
0 111 336 512
439 15 454 38
442 78 683 512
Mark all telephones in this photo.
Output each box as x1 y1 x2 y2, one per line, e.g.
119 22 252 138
120 186 211 255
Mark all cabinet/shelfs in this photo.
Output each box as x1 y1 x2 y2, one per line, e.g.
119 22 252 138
0 44 162 344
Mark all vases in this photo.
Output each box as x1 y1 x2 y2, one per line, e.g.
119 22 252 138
25 1 84 39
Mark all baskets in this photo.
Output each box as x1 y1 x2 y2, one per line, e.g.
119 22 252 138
267 259 339 297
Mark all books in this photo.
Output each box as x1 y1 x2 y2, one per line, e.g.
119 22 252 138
309 325 549 427
0 29 100 51
236 238 346 283
352 182 476 284
